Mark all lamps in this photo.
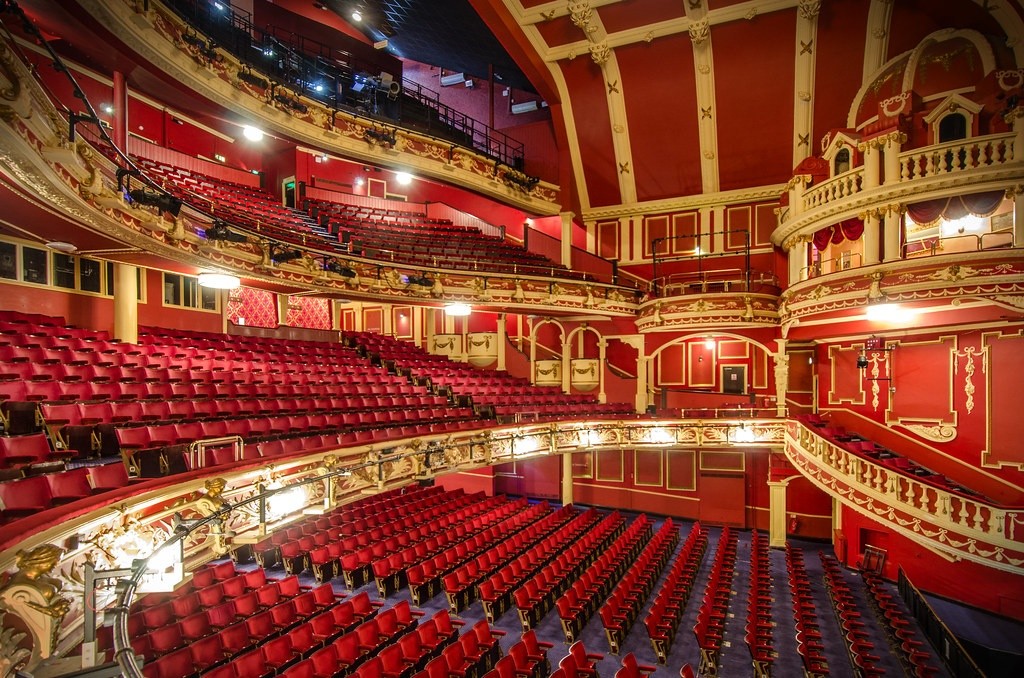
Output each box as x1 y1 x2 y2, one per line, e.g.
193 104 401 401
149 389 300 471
204 219 249 244
271 242 304 263
366 72 400 102
408 271 437 286
128 186 185 217
444 305 472 316
704 336 717 352
325 254 358 278
46 242 77 254
195 272 240 290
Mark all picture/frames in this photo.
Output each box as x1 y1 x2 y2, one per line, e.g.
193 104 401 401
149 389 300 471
840 250 852 270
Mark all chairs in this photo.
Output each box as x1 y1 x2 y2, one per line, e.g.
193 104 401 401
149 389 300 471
92 141 596 280
1 308 960 678
